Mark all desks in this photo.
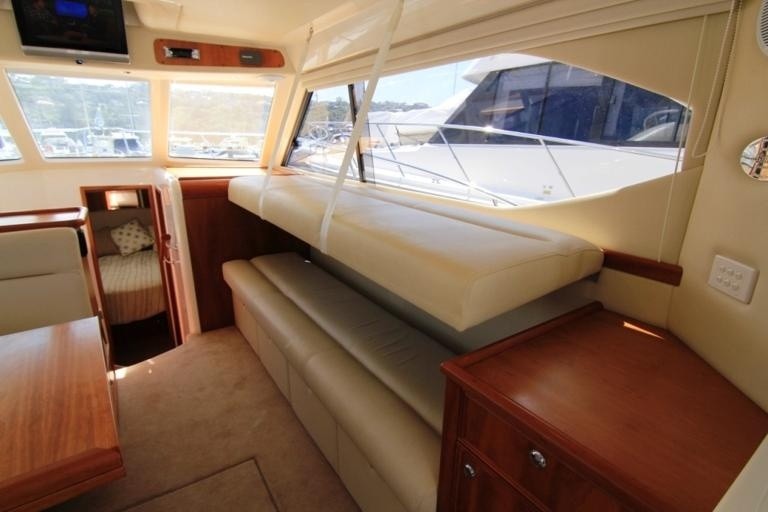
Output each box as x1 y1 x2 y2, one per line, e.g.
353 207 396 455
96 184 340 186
1 315 130 510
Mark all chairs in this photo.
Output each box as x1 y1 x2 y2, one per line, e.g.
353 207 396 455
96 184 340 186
1 225 95 338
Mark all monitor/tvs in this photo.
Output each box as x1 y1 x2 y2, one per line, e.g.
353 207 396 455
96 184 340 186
10 0 132 64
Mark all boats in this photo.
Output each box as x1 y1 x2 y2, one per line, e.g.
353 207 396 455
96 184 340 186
310 52 690 205
32 129 142 155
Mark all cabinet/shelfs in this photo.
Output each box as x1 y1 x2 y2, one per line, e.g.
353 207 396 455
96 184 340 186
434 303 768 512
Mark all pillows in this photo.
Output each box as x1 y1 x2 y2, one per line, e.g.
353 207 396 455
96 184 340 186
93 218 157 258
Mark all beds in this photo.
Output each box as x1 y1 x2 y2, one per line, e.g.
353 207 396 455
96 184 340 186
221 252 460 510
227 172 602 333
88 207 166 327
